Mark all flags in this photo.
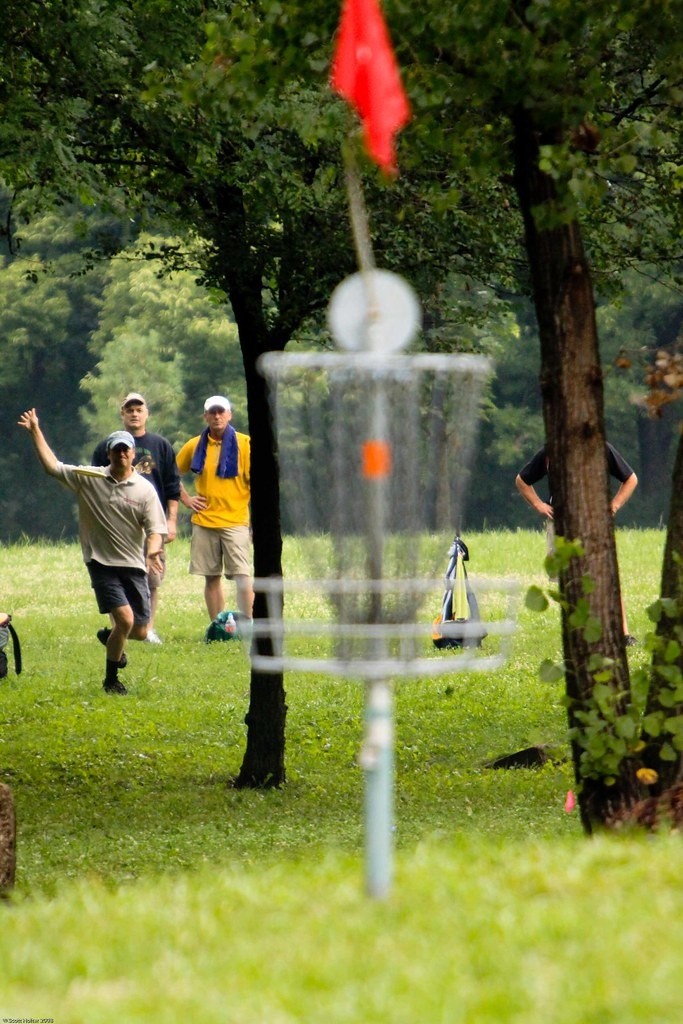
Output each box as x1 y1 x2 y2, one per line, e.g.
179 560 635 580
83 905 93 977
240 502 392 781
333 0 410 173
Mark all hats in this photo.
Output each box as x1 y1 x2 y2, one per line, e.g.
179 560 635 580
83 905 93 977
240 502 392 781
106 431 136 449
121 393 146 406
204 396 230 410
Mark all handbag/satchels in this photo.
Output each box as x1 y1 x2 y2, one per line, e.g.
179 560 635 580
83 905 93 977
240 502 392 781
204 609 253 645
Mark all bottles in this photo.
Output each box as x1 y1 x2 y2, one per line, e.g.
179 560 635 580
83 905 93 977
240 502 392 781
225 613 237 634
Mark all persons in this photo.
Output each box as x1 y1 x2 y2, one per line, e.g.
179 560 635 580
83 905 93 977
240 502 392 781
89 392 181 647
515 436 639 648
175 396 253 621
18 407 170 694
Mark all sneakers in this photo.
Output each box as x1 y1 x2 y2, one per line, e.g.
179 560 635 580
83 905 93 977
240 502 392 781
98 628 128 668
102 676 128 697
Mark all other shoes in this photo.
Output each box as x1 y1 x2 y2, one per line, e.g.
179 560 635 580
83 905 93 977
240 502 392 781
147 632 163 646
